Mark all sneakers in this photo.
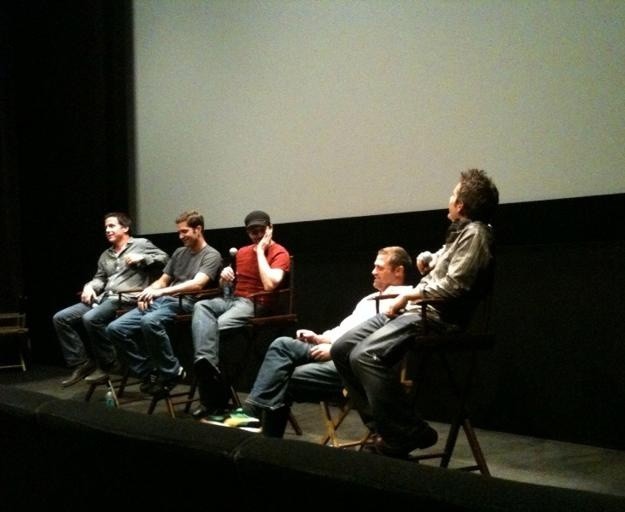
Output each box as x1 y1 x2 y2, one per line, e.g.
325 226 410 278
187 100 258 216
140 367 186 396
202 407 263 433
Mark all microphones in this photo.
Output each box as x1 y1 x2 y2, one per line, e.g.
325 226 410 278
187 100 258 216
228 247 238 285
419 250 433 275
79 293 98 308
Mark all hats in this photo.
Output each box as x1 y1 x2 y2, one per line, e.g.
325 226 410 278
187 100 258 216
244 210 270 227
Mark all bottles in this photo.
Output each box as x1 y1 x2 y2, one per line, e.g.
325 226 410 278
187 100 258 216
223 283 231 298
105 389 115 406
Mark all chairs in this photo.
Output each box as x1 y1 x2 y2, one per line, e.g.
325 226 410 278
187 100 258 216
74 222 499 479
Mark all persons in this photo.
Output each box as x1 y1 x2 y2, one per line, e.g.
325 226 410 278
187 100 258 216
329 166 503 457
190 208 297 420
243 244 441 437
105 209 223 396
50 210 171 388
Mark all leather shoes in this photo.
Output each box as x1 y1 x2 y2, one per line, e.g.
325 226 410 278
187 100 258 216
85 369 110 384
61 361 95 387
361 422 438 454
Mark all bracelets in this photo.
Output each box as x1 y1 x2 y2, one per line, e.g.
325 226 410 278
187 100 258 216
419 283 428 299
